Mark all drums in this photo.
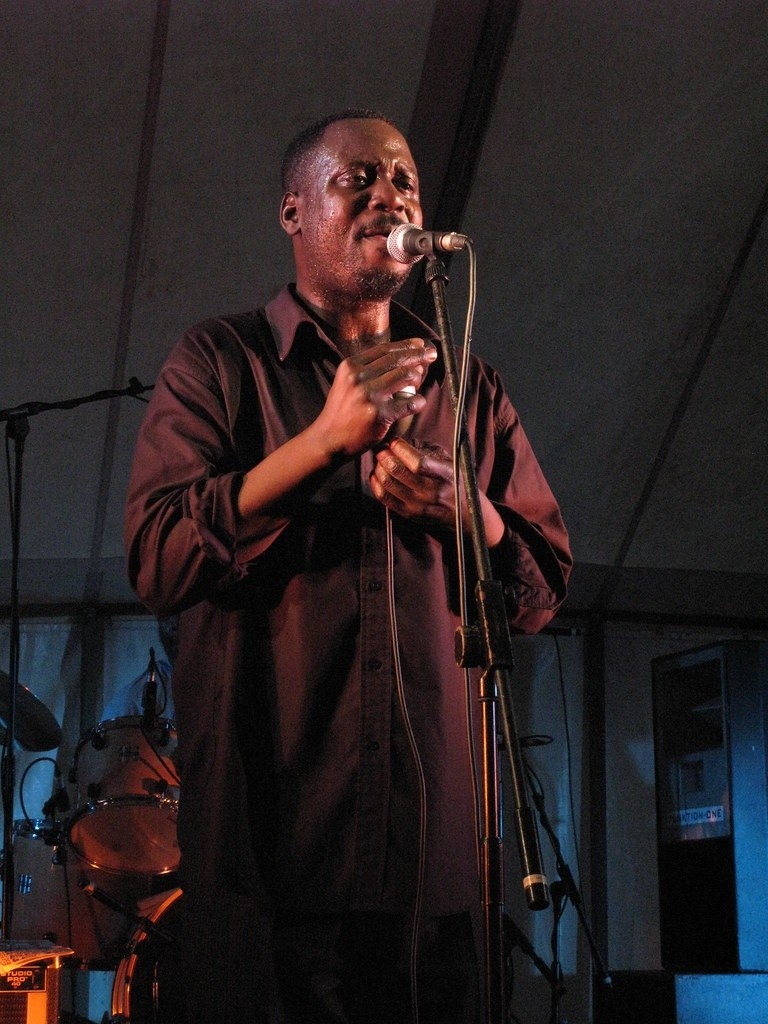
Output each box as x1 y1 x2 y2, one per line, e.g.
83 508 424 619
112 887 183 1024
10 818 128 970
68 714 183 876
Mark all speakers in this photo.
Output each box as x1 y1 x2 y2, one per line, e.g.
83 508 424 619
652 637 768 975
608 970 768 1024
0 956 60 1024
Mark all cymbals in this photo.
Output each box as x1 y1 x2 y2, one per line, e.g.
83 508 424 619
0 667 63 753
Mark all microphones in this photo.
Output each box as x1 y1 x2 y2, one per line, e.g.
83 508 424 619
141 657 157 732
388 223 470 263
54 768 70 813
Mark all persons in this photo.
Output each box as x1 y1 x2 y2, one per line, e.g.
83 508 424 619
124 107 572 1024
